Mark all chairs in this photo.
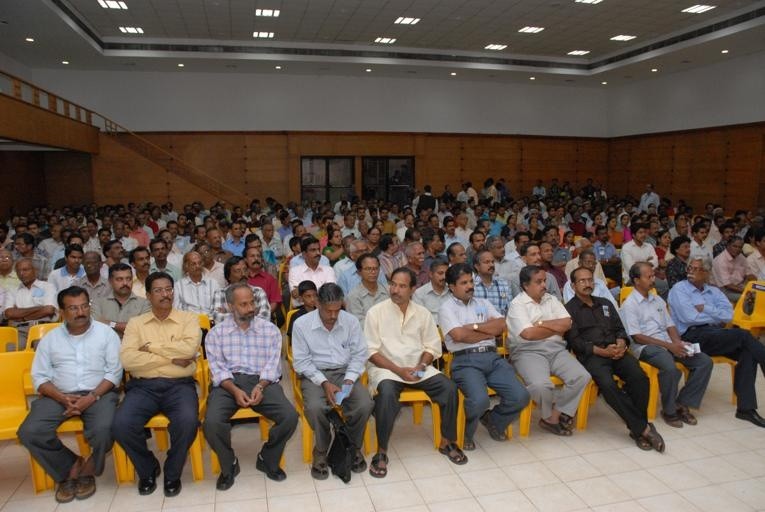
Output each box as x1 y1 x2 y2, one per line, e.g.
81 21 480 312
363 364 444 456
569 343 657 431
203 358 287 476
0 328 47 494
109 314 209 489
620 285 690 423
21 323 128 494
285 310 372 464
721 280 765 338
500 330 588 439
665 289 739 408
439 325 513 451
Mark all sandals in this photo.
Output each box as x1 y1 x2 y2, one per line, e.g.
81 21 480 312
645 422 665 453
439 443 468 465
369 451 389 478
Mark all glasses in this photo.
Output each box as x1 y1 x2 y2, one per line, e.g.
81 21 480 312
687 266 708 272
575 278 593 284
62 302 91 311
0 256 11 262
149 287 175 296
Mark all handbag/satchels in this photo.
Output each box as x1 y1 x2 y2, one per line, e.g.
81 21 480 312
329 414 357 483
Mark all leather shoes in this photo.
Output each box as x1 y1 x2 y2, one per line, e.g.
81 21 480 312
737 407 765 429
481 410 506 441
164 469 182 496
537 417 571 436
560 412 572 427
629 430 652 450
660 410 683 428
216 458 240 490
676 401 698 425
256 453 287 481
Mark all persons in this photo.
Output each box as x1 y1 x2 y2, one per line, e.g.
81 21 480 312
166 220 187 257
621 224 659 287
234 190 306 223
223 222 245 258
176 215 195 247
592 226 617 259
465 231 486 260
158 230 184 271
563 250 619 313
420 362 428 370
243 235 277 281
423 234 448 268
564 237 608 287
512 233 531 256
255 224 282 258
416 176 543 233
60 226 75 245
96 228 115 261
242 248 282 313
14 225 28 234
11 234 51 281
508 243 563 305
411 262 451 327
288 280 319 350
300 233 315 248
560 230 576 250
404 229 421 253
337 240 388 297
708 235 757 315
438 263 531 451
0 225 13 249
469 250 513 316
617 342 628 353
472 323 479 331
37 224 65 280
538 242 568 289
0 248 22 327
668 198 765 223
201 283 299 491
665 221 699 260
148 238 182 281
712 222 735 260
66 234 84 248
543 228 560 253
292 282 372 480
292 223 307 237
653 229 671 260
537 321 544 326
447 242 477 283
191 242 229 288
205 226 233 264
666 236 691 296
332 235 358 281
343 254 391 330
485 236 518 287
192 202 205 223
617 261 714 428
94 263 152 338
667 258 765 428
257 384 265 392
746 231 765 284
144 342 152 348
376 233 404 280
112 272 203 497
363 268 468 478
183 204 196 225
562 267 665 455
120 223 137 252
687 222 710 258
100 240 135 281
397 241 432 299
27 223 40 234
283 236 301 281
78 227 98 252
363 228 382 253
311 198 364 230
129 246 153 300
202 214 220 232
210 201 227 219
543 176 590 228
322 230 346 267
364 198 415 228
108 321 118 329
506 264 591 437
192 224 208 247
214 253 270 324
4 200 177 223
236 220 247 236
16 285 124 504
48 244 86 292
173 250 221 326
90 391 100 402
288 238 337 321
5 258 58 352
590 182 668 223
69 253 110 319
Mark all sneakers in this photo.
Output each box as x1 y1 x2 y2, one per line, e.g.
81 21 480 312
350 450 367 473
311 461 329 480
463 438 475 450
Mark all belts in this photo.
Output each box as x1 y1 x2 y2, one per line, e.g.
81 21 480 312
232 371 248 376
320 368 340 372
454 345 497 354
16 321 48 328
136 375 176 381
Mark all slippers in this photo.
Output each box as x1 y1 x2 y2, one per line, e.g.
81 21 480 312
56 478 75 503
138 465 160 495
75 471 96 499
